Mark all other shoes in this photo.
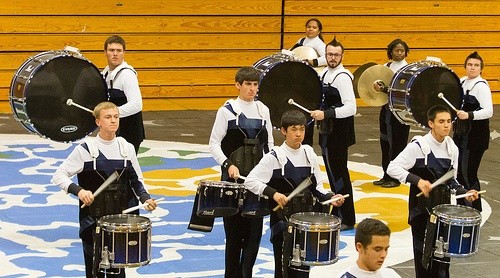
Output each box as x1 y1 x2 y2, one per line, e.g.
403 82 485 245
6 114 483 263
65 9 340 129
340 223 354 231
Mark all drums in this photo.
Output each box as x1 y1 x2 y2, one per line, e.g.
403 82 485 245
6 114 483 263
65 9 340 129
390 60 463 128
254 54 324 130
288 212 340 266
432 204 482 258
9 50 109 144
95 215 151 268
241 183 271 217
197 182 238 218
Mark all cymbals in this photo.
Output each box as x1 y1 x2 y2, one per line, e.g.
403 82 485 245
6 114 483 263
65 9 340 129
349 63 395 107
287 43 319 62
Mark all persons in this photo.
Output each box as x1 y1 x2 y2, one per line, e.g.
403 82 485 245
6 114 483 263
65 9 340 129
372 38 410 189
387 105 480 278
340 218 401 278
244 110 344 278
452 52 494 212
102 34 145 157
310 35 358 231
209 66 275 278
52 102 157 278
296 17 329 146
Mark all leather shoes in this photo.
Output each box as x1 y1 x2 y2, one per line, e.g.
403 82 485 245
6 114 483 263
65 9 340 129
382 181 400 187
373 176 386 185
